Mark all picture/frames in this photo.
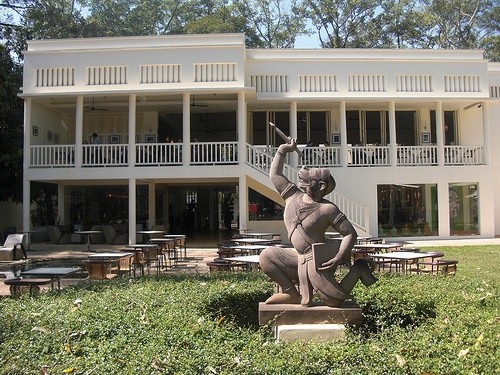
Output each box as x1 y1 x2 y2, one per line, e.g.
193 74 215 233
32 126 60 143
332 133 341 144
89 134 155 143
422 132 430 143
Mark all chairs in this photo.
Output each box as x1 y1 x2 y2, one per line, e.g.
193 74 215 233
206 235 459 276
0 226 187 299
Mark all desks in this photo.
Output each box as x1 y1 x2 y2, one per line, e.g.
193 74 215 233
136 231 166 243
326 237 371 240
354 244 402 251
125 244 158 247
149 238 174 241
74 231 102 253
89 253 132 281
17 231 41 251
324 232 340 238
370 252 438 276
20 267 83 292
222 229 275 271
164 235 185 237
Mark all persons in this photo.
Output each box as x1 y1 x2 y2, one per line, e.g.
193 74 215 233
259 137 379 306
372 143 385 163
353 141 365 158
302 141 315 164
93 132 102 163
324 140 332 160
434 188 460 218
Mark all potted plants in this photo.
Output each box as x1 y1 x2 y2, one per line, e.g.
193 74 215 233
30 208 49 242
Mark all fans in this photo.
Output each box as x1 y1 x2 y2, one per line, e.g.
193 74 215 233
85 97 109 112
190 94 209 107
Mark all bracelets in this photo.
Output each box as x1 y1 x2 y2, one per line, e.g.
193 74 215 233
336 253 342 258
276 151 286 156
